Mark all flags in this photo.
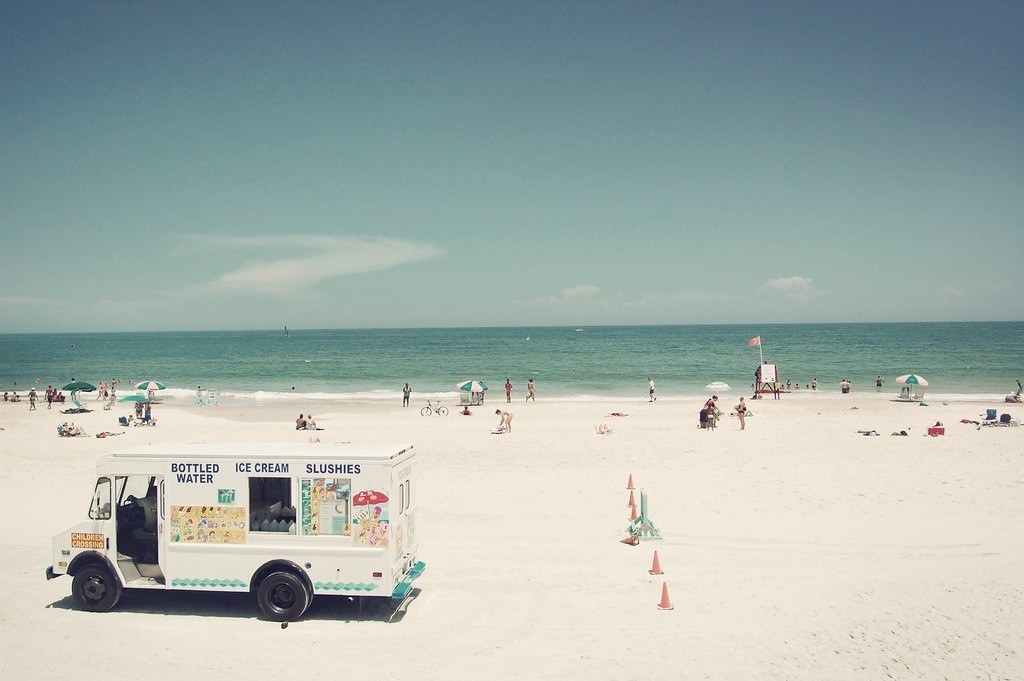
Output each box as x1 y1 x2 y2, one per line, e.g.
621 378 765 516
748 336 760 347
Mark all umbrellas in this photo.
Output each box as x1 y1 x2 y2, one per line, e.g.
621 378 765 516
62 381 97 400
117 396 149 418
135 381 166 399
456 380 488 406
703 381 731 408
896 375 928 401
353 490 389 519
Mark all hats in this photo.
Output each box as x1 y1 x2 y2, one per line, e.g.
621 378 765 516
31 387 35 391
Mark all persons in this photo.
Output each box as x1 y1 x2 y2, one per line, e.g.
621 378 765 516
96 378 118 411
840 379 851 393
122 402 157 427
700 395 752 430
781 377 818 391
648 378 657 402
4 391 21 402
70 377 77 401
1005 380 1023 402
459 406 472 415
126 486 157 564
58 422 86 436
26 385 65 410
526 379 537 402
505 379 513 403
296 413 317 430
876 375 885 387
495 409 513 433
95 431 126 439
403 383 411 407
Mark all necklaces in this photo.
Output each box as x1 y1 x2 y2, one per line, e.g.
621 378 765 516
143 498 154 507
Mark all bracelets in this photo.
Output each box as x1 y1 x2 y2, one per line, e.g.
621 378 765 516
148 533 156 544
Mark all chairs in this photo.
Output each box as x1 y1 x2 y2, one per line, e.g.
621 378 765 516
912 388 924 400
896 387 910 399
194 387 217 406
460 394 470 404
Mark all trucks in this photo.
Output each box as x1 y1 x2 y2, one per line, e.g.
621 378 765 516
46 441 427 620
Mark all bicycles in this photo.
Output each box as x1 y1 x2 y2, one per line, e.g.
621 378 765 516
420 400 448 417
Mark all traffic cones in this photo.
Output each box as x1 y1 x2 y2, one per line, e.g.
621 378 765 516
649 552 663 576
628 507 638 523
657 580 674 612
625 474 635 488
621 534 638 546
626 490 637 507
